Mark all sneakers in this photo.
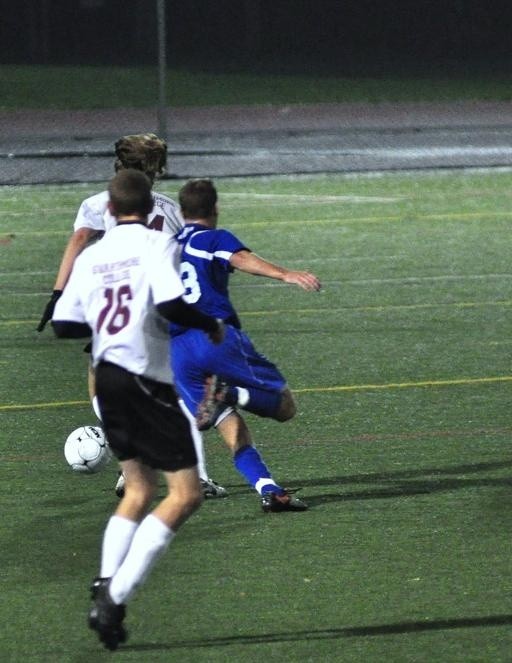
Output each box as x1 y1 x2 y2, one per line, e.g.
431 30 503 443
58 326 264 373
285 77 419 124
199 477 226 497
195 373 230 430
90 598 128 641
95 584 126 651
115 470 124 497
260 486 308 510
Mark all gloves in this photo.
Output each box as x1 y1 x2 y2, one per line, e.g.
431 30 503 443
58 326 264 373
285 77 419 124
36 290 62 331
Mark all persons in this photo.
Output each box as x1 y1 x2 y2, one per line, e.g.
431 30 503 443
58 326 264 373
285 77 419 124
35 131 228 501
168 177 327 512
50 166 227 651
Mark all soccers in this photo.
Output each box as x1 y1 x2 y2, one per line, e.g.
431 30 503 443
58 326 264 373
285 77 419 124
64 425 112 472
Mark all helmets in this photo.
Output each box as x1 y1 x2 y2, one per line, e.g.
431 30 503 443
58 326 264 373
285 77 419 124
114 133 168 180
